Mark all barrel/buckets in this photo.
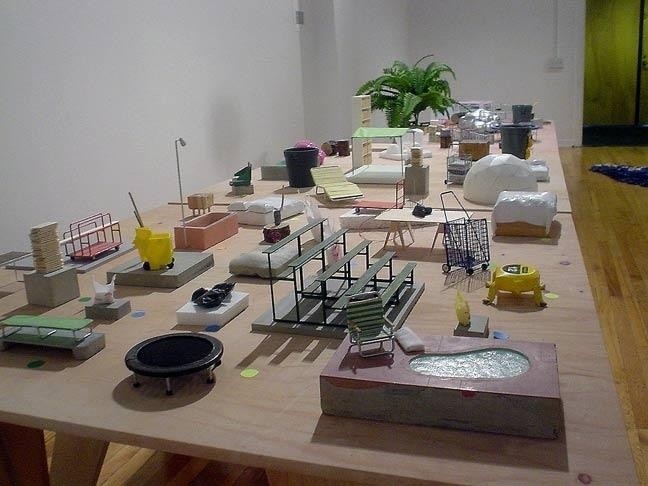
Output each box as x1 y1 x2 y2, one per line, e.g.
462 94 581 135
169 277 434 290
512 105 535 122
501 124 531 159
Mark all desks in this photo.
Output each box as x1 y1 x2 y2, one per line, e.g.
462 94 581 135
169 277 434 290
1 120 640 486
374 209 475 250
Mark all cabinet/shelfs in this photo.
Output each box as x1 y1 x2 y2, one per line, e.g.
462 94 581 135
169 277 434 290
353 95 372 169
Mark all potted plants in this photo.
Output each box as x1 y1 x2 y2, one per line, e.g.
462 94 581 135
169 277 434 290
355 53 472 147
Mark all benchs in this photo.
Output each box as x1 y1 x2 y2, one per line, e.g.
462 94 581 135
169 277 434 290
261 216 417 328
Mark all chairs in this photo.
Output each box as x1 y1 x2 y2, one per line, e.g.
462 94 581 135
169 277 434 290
309 165 364 202
346 291 395 358
